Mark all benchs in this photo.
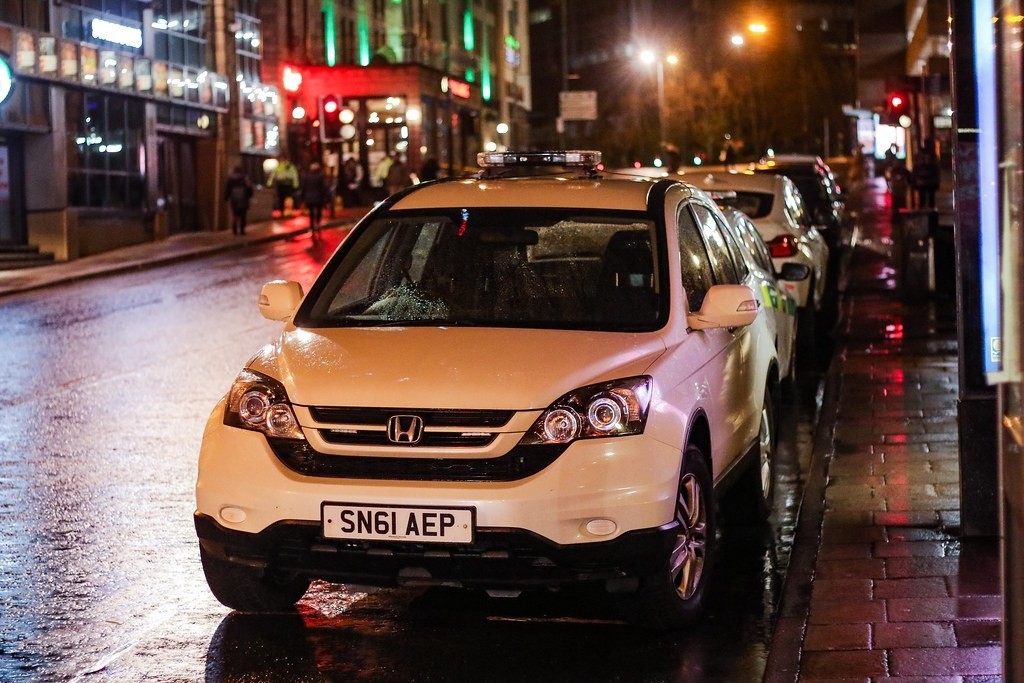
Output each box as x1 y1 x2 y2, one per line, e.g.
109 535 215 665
529 258 603 323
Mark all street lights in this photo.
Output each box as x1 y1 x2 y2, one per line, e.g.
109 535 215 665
641 50 678 141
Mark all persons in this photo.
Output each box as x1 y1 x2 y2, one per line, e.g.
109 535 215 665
885 138 940 209
300 169 331 232
337 151 438 210
225 167 254 237
266 154 298 218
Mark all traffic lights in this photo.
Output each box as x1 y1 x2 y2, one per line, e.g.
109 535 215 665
322 95 341 139
886 89 910 127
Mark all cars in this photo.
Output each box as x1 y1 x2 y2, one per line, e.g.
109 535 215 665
660 154 844 396
194 151 780 623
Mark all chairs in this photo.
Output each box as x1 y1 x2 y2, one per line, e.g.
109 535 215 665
592 228 655 321
